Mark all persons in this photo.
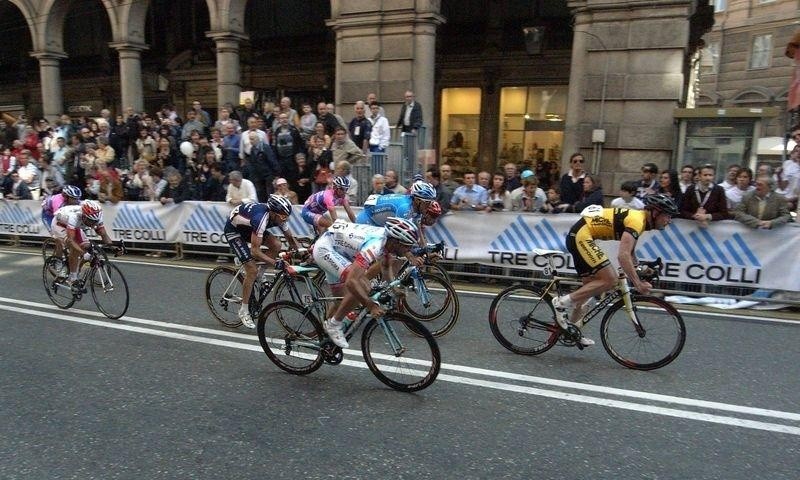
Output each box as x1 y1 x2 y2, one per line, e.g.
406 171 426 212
302 177 444 348
610 124 800 303
396 91 423 171
2 90 390 261
225 193 304 330
370 151 604 289
551 202 674 346
50 201 125 294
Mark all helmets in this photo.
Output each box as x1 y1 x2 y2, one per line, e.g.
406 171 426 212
63 185 82 198
644 194 681 215
81 200 102 221
384 217 420 246
411 180 442 216
266 194 292 216
333 175 351 190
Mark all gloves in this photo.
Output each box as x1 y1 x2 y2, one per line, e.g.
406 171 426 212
274 260 285 270
83 253 93 263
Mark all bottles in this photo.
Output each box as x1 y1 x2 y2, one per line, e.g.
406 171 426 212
461 203 476 210
580 294 601 309
533 195 543 210
78 261 91 280
260 276 269 291
522 196 531 210
341 311 357 330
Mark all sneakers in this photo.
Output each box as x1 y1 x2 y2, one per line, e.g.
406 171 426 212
66 277 81 295
579 337 595 345
552 296 568 330
323 319 350 348
239 309 256 329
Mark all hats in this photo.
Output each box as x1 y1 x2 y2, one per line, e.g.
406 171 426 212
276 178 287 185
521 170 534 179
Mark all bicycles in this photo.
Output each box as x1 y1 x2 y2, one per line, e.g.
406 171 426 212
42 235 112 286
258 263 441 394
489 247 687 371
42 239 130 320
206 229 460 339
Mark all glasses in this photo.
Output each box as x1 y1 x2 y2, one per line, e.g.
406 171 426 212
574 160 584 163
